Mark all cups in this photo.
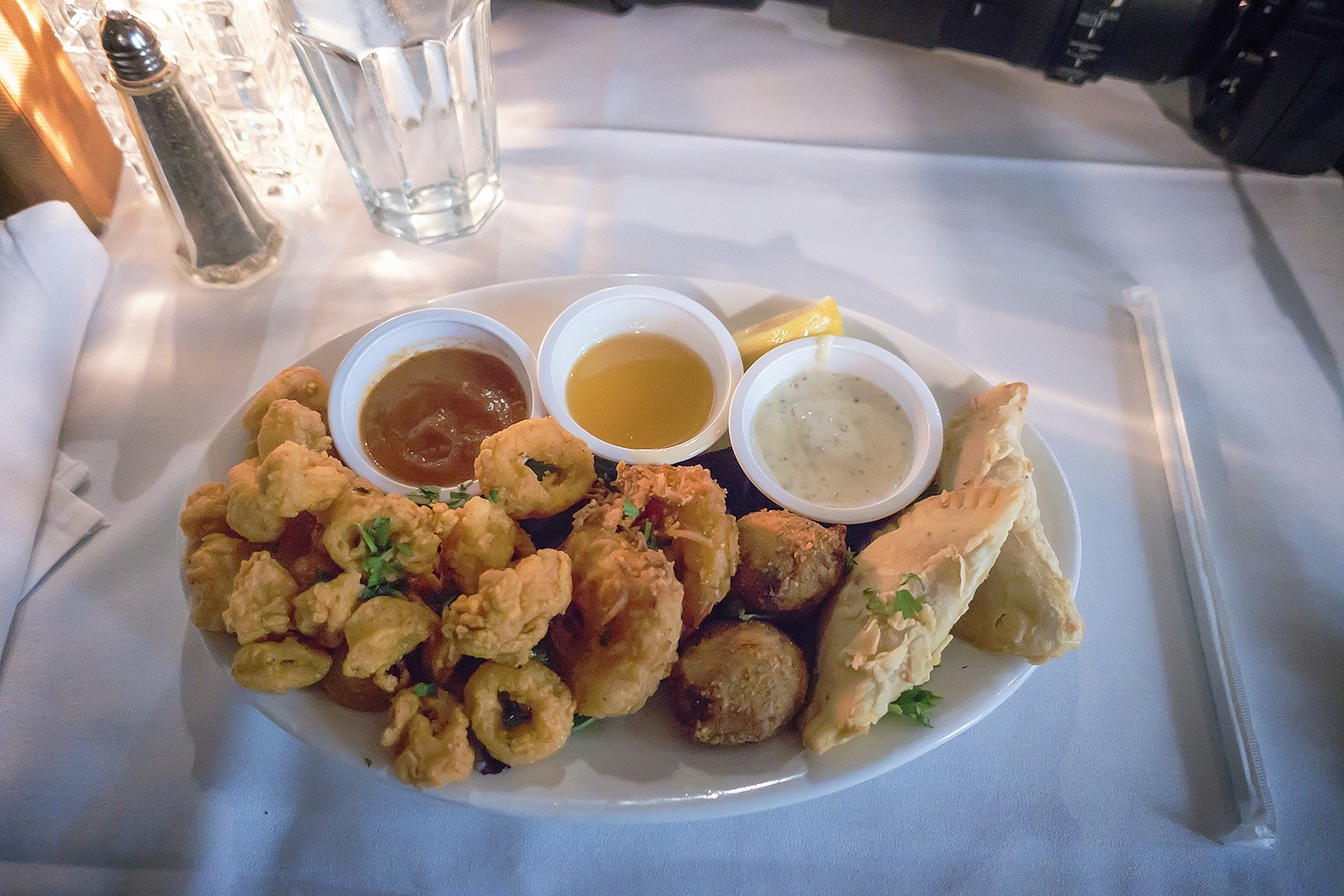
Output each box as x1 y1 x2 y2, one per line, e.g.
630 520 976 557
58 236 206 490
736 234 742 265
274 0 505 247
38 0 330 211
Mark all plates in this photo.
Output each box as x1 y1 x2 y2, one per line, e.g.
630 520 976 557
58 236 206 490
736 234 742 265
176 273 1091 826
